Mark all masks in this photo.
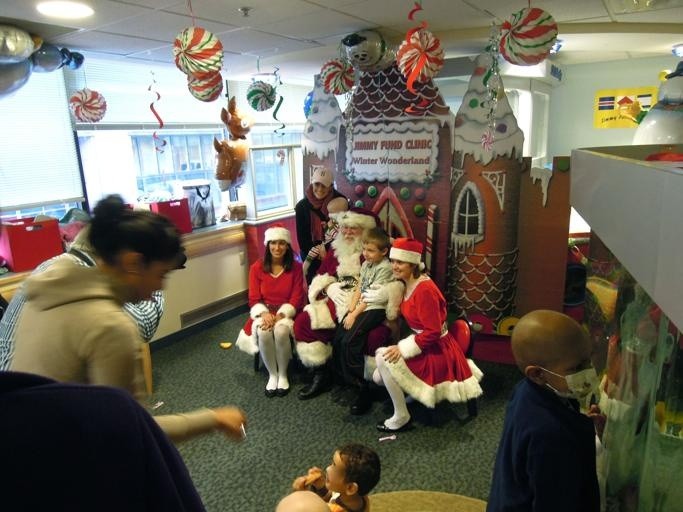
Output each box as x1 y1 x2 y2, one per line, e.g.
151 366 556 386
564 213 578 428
533 364 598 399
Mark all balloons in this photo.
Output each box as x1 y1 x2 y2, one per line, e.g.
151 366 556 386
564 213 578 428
339 28 396 75
220 97 249 139
213 139 249 191
0 69 29 95
1 27 34 66
31 43 84 74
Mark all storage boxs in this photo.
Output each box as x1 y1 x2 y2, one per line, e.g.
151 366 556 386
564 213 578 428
126 197 193 233
0 215 65 272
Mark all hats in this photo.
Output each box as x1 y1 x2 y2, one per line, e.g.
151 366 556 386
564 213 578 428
264 223 293 245
312 168 337 187
343 207 382 228
390 238 424 264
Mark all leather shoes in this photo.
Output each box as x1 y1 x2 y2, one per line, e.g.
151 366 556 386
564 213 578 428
266 376 276 397
277 371 290 398
377 416 416 433
297 368 332 399
350 380 373 416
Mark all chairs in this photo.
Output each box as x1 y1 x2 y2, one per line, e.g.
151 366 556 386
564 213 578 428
423 315 480 418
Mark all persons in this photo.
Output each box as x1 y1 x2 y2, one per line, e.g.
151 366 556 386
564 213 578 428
296 170 349 260
10 193 246 442
2 222 165 379
376 239 485 433
292 444 380 512
487 308 606 511
293 212 378 399
308 198 347 263
248 227 304 395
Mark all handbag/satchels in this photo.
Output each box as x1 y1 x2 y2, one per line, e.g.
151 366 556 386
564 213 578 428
184 182 216 229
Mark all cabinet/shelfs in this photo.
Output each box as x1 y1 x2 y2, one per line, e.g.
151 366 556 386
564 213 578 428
0 220 249 346
